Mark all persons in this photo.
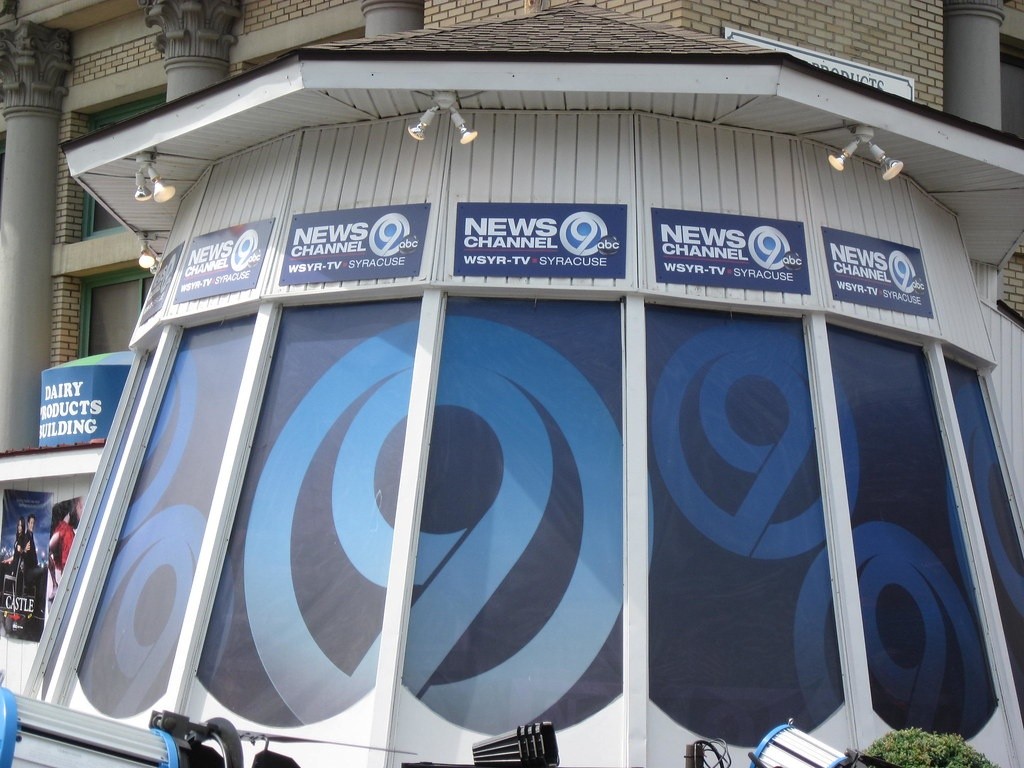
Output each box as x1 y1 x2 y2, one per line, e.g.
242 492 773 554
20 514 46 620
1 514 25 592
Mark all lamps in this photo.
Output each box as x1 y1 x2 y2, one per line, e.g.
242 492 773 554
407 106 438 140
450 107 478 144
135 164 152 201
869 139 903 181
147 166 175 203
828 138 859 170
